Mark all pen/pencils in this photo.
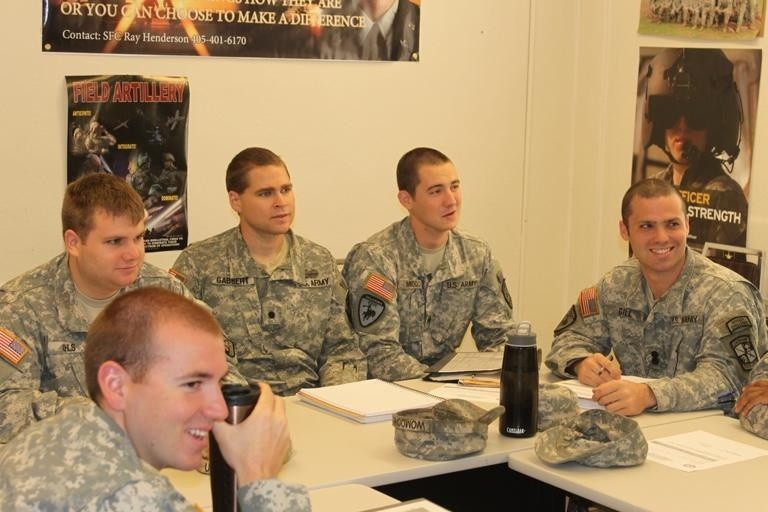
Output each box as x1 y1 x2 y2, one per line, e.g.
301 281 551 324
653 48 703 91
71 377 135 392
598 355 613 375
471 373 475 378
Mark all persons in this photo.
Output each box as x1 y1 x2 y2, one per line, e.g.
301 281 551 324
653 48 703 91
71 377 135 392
647 48 748 251
319 0 419 61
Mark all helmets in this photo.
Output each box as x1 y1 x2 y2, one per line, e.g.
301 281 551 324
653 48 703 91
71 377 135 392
641 48 741 164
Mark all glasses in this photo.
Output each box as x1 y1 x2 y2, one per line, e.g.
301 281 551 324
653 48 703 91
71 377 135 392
660 107 707 129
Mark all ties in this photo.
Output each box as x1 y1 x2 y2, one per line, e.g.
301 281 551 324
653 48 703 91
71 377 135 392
361 24 380 59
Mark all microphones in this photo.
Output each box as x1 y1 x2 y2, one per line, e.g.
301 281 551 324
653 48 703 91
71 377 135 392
680 138 739 165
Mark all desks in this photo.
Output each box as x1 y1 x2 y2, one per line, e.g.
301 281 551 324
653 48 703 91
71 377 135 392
165 370 768 511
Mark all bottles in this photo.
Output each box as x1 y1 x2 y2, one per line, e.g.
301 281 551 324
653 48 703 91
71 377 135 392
208 383 261 512
499 319 540 438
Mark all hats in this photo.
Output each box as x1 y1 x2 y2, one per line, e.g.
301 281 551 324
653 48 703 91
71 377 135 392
535 383 648 467
392 398 506 461
